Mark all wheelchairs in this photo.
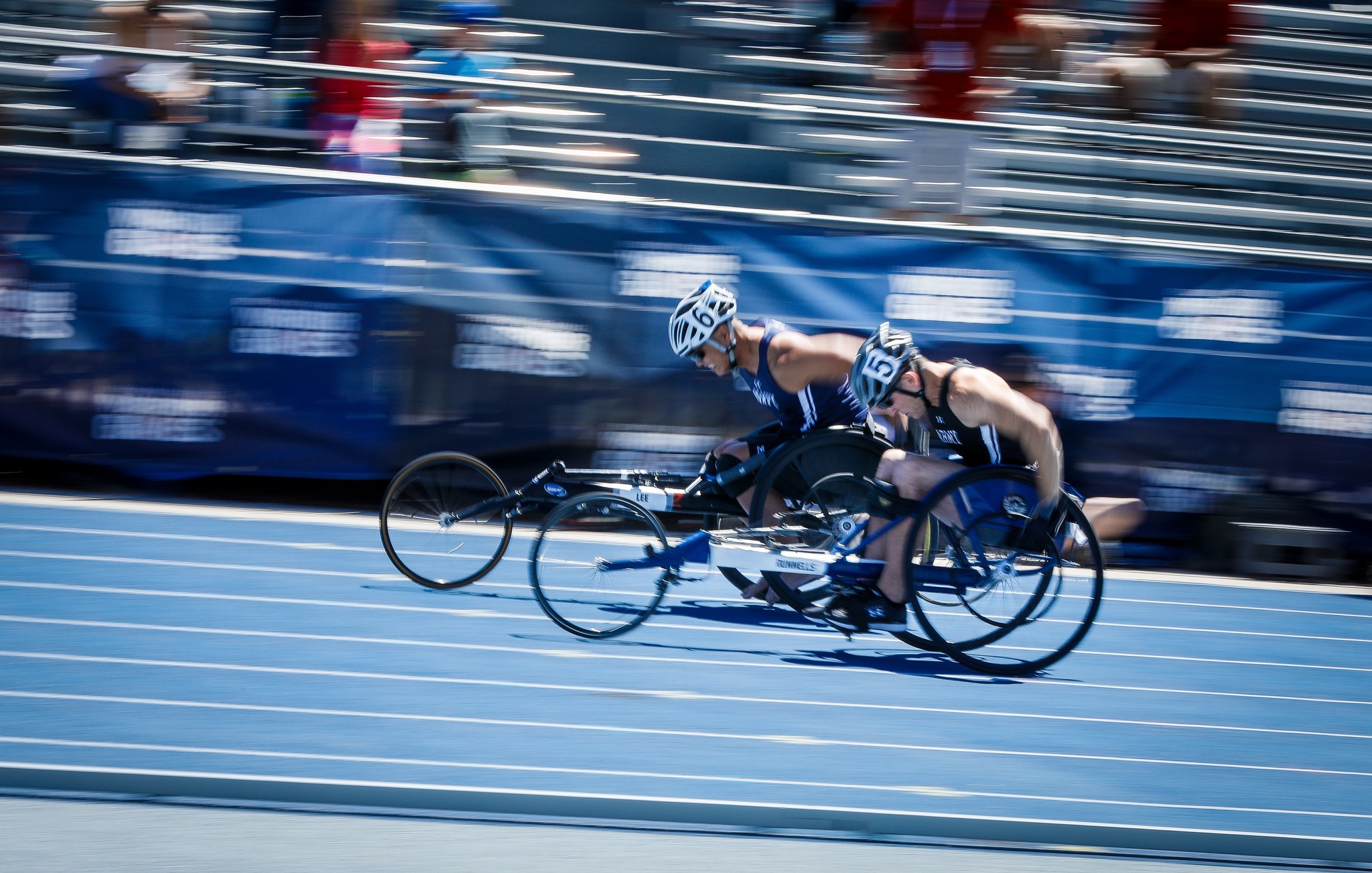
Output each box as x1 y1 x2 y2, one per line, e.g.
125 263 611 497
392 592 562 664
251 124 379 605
528 444 1106 681
373 417 940 619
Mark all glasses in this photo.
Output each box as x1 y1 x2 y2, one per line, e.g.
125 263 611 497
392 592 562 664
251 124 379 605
687 347 705 363
877 366 910 410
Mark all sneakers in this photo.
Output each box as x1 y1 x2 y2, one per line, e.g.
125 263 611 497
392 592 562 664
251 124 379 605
821 588 906 631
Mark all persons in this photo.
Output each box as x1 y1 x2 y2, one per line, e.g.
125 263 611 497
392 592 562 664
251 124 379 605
802 322 1065 633
668 279 909 607
796 0 1244 221
44 0 547 187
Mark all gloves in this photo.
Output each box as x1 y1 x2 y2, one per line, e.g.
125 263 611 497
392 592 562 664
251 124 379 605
1019 512 1052 552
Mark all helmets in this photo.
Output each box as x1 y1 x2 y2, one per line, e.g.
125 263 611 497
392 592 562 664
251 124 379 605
668 280 737 358
851 322 912 410
